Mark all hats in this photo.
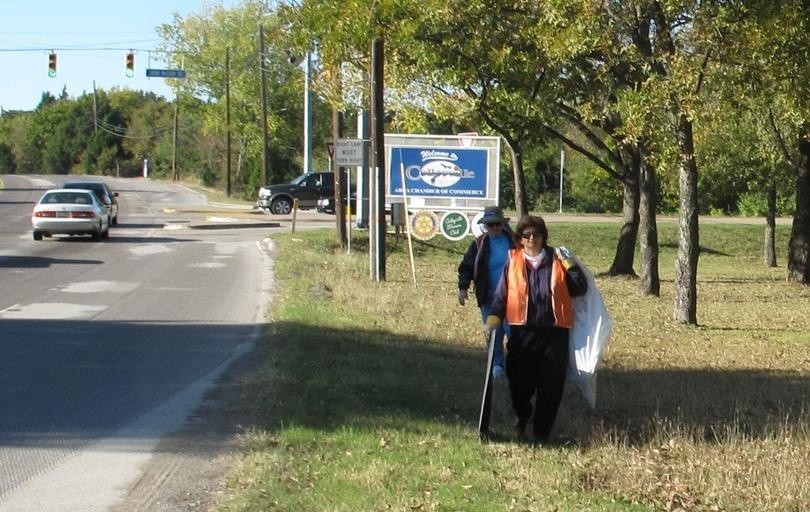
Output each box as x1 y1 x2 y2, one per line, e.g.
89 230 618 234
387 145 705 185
477 206 510 224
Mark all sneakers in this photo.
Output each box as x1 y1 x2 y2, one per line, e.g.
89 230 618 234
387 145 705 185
493 365 505 379
513 405 533 433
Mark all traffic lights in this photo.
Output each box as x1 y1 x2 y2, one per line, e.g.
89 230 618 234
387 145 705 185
48 54 57 78
126 54 135 77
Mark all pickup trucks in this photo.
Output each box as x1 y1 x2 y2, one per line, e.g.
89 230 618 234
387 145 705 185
256 172 356 215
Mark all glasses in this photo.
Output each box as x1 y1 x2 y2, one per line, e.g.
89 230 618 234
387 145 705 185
485 223 503 227
522 230 543 238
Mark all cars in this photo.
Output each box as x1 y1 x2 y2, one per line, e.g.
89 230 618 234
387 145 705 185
64 181 119 226
32 188 110 240
316 192 357 214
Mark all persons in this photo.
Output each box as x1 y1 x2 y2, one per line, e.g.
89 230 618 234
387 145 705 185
482 213 589 446
454 205 520 381
